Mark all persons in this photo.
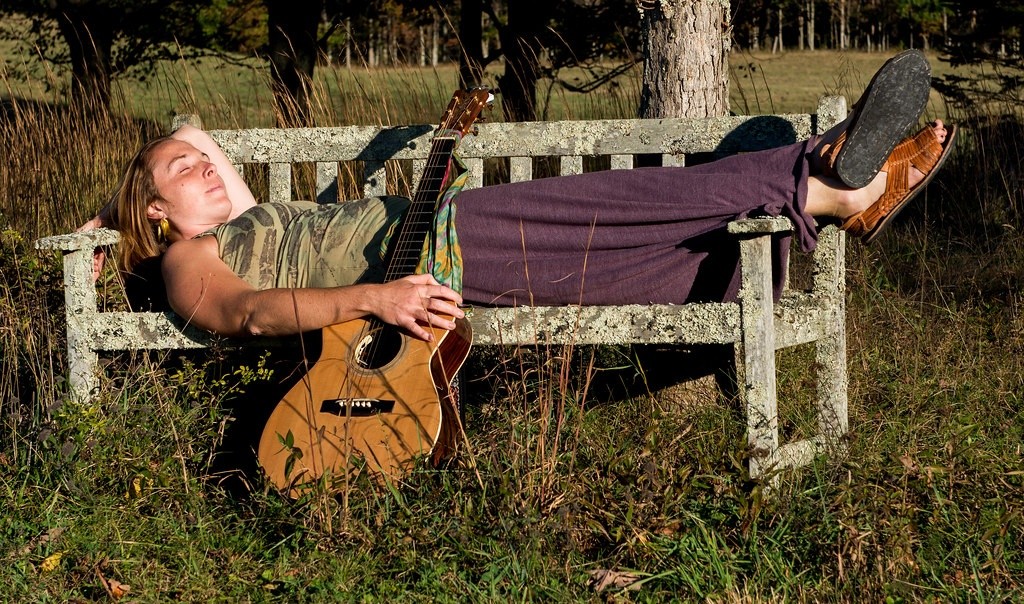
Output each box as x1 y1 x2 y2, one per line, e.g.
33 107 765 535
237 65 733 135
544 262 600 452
79 48 958 337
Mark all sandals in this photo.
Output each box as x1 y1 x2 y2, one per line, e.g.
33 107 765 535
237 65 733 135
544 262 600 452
838 122 958 244
821 48 932 189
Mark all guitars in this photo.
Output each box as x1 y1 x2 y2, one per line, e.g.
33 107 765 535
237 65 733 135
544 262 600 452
254 82 496 509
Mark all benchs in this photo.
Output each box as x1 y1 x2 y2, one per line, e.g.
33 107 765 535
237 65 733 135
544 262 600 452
38 94 854 492
